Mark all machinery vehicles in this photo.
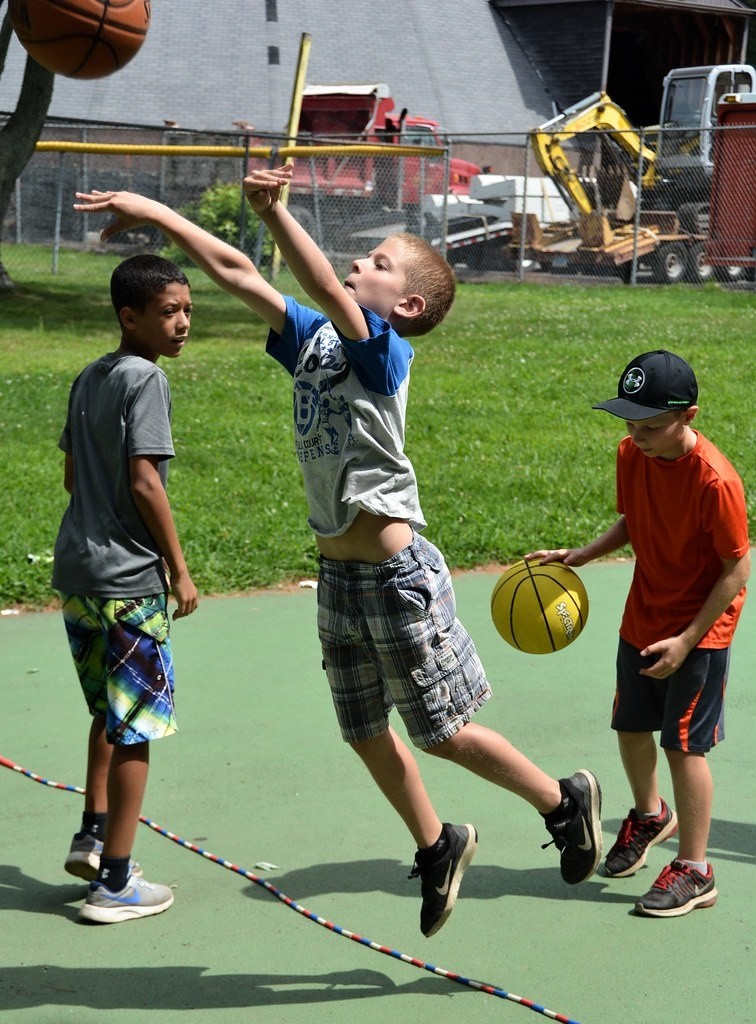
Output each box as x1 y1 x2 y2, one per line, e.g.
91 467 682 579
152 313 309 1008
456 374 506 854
503 64 756 281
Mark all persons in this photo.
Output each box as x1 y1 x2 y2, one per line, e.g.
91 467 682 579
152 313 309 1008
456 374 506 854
74 165 602 938
523 350 751 918
48 255 198 924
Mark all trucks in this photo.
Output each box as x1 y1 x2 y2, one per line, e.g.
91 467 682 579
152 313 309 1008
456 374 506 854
264 84 491 253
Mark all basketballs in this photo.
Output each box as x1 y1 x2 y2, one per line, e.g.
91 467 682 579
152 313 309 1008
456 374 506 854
8 0 150 82
488 559 588 657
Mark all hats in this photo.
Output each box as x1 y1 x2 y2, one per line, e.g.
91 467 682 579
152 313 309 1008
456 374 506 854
592 350 698 420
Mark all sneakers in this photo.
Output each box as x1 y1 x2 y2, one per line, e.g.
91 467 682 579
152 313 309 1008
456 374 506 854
541 769 604 885
605 797 677 878
407 822 477 938
636 857 718 917
77 863 174 922
65 831 144 882
